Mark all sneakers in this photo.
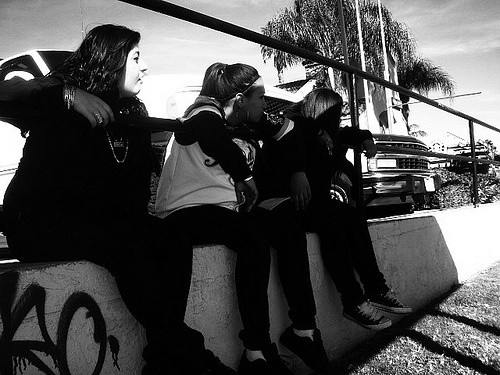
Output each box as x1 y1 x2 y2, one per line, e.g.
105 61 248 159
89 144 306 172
343 301 392 330
369 291 412 313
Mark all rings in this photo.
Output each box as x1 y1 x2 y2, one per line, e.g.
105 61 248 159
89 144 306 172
95 113 102 124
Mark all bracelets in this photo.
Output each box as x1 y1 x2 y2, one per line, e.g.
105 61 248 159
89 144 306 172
244 176 253 182
64 84 75 110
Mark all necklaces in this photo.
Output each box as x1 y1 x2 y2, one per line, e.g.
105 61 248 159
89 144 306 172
105 127 129 163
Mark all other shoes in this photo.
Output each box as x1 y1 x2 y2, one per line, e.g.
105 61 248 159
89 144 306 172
237 342 292 375
279 326 335 375
141 350 239 375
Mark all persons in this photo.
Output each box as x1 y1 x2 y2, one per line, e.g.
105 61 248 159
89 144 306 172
0 25 236 375
252 89 412 331
154 63 311 375
